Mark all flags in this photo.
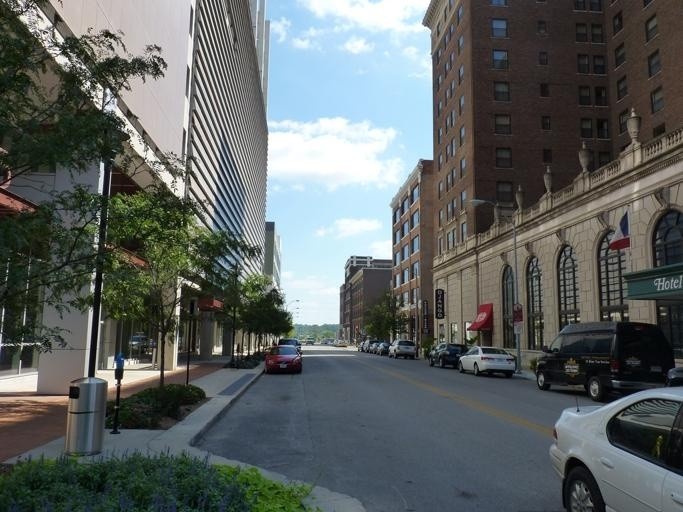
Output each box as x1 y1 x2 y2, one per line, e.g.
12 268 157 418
609 211 630 252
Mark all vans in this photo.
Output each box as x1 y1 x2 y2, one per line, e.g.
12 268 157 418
535 321 675 402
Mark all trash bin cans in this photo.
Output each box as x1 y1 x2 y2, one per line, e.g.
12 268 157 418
66 377 108 456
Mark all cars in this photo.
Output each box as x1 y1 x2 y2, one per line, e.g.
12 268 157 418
128 333 148 352
458 346 516 378
302 337 315 345
548 366 683 512
428 343 469 368
357 339 417 359
320 338 348 348
265 335 303 374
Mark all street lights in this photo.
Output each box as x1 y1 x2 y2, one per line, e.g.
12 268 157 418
286 299 299 318
469 199 522 374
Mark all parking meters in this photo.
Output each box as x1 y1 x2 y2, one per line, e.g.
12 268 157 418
109 352 125 434
